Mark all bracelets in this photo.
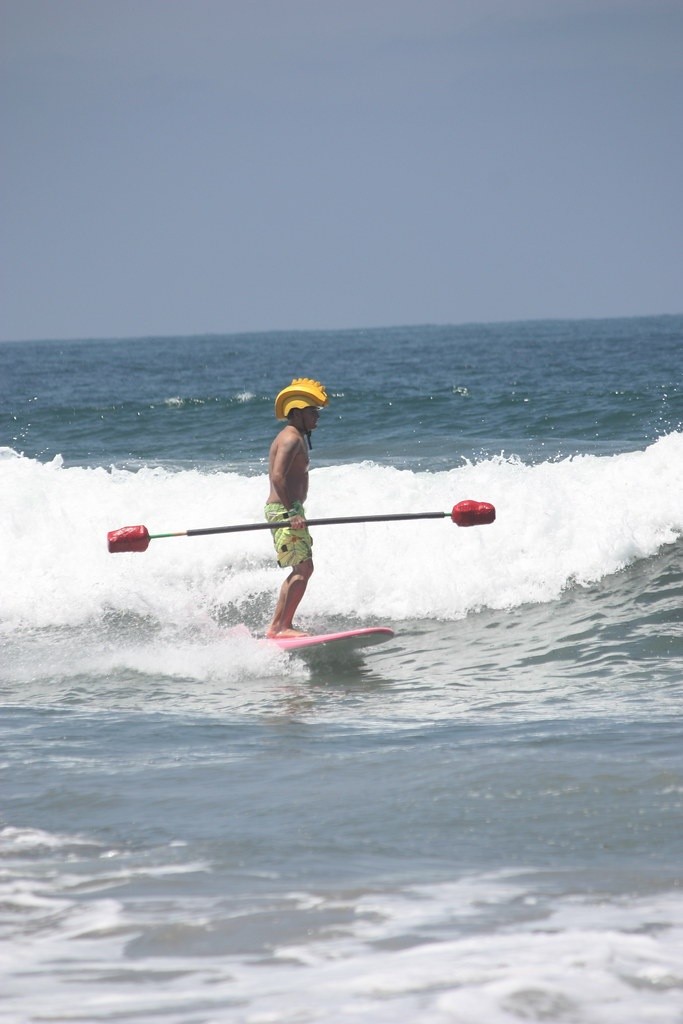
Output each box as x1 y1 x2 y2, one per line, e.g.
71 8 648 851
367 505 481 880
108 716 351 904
287 509 298 517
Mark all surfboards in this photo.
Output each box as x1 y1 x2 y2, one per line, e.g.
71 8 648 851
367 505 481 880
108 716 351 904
269 627 395 654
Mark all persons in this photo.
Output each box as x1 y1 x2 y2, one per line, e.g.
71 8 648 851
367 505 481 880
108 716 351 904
265 396 319 638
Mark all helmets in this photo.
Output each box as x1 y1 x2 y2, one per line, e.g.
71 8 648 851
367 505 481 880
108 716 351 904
275 378 328 418
